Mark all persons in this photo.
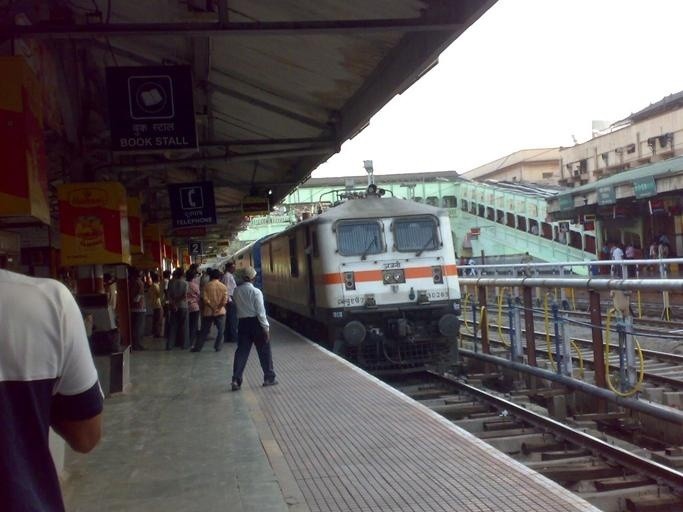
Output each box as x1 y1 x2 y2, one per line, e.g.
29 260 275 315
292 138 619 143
103 271 119 326
530 222 538 235
2 268 105 512
228 265 279 393
557 222 567 244
127 261 238 353
455 254 477 278
518 250 533 277
600 231 670 279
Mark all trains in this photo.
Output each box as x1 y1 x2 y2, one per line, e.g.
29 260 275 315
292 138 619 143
213 184 462 376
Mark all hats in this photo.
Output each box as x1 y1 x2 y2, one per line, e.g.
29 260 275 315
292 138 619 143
242 266 257 280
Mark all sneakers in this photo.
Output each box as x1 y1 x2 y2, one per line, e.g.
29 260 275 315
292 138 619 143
230 378 240 391
165 343 223 352
262 378 278 387
